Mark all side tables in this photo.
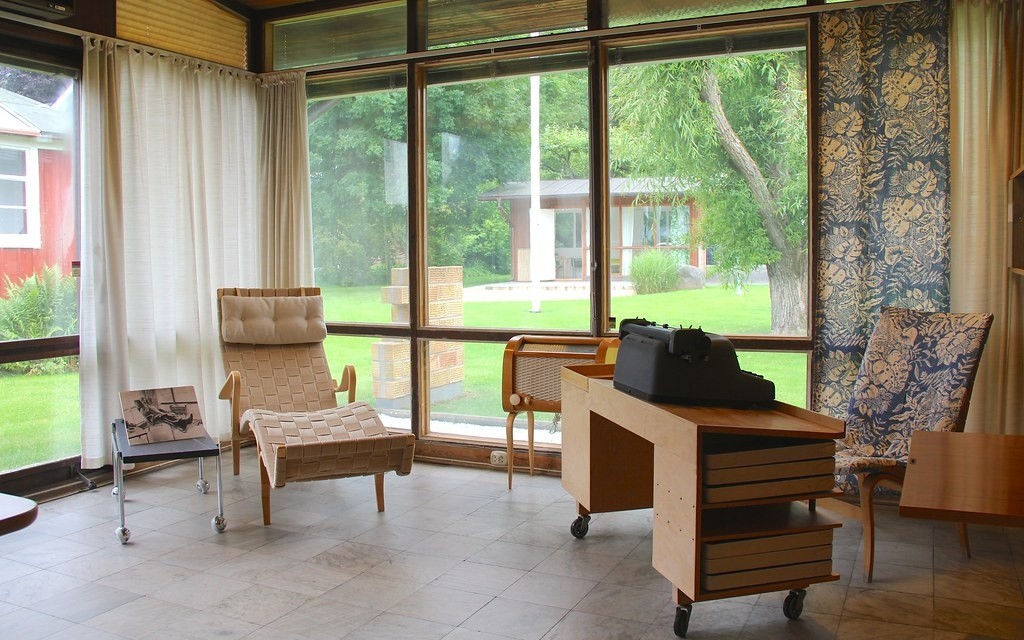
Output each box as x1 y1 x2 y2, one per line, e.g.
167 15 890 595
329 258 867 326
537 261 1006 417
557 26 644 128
110 417 227 547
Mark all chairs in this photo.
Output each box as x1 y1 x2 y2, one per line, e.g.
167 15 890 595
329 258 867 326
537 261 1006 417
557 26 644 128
216 287 415 527
829 306 997 584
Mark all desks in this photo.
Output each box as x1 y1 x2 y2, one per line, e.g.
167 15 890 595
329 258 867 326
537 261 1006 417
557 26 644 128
1 491 40 540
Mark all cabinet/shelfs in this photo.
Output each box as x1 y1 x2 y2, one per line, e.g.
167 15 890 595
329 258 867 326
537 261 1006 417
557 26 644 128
559 361 845 632
